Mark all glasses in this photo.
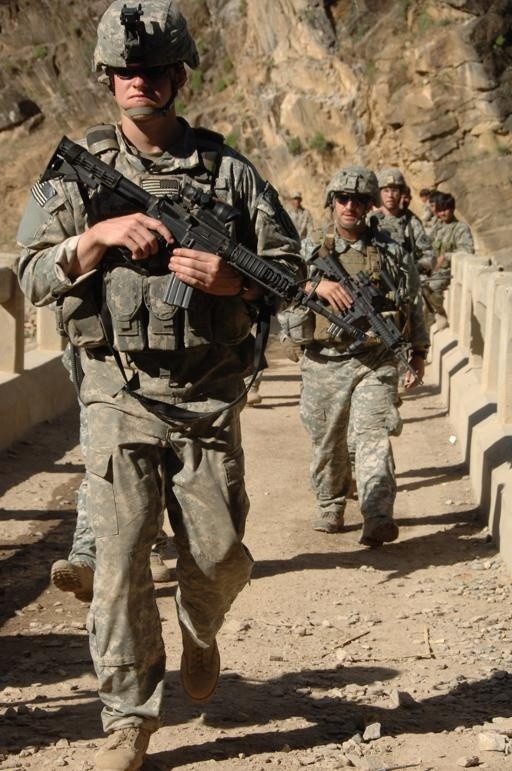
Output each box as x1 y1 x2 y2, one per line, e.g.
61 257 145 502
116 69 168 80
335 195 365 204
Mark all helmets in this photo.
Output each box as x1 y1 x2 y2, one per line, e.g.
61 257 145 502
377 169 404 188
324 168 381 211
93 0 201 74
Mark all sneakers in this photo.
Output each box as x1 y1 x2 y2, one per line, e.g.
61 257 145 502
149 553 169 582
246 390 261 407
181 619 219 700
54 559 93 602
93 726 148 769
314 512 344 532
359 522 399 547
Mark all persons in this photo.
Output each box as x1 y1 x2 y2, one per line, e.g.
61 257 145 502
365 170 475 326
16 0 309 771
287 191 314 237
276 166 431 547
51 320 170 601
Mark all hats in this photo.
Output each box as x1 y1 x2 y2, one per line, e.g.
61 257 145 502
290 192 301 199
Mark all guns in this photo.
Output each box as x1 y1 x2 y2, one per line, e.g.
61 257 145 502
39 134 369 341
381 270 410 312
305 245 423 385
